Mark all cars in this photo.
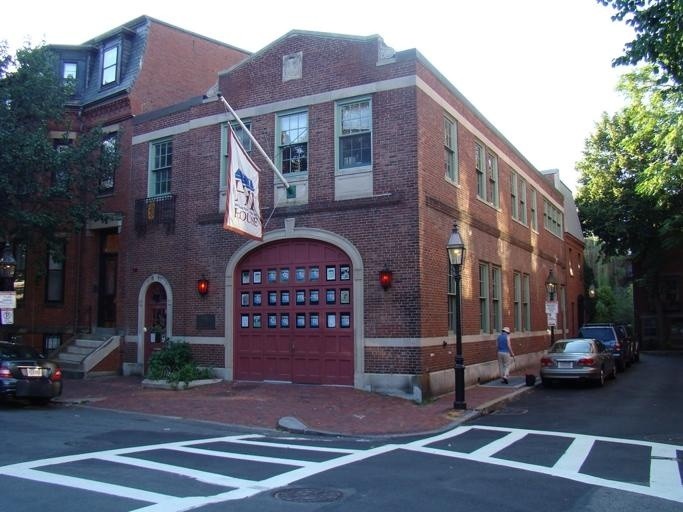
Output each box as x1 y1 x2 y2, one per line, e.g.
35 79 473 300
0 344 64 401
540 339 616 384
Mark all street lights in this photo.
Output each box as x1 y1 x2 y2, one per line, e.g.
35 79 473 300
0 243 16 343
445 219 466 410
544 269 559 346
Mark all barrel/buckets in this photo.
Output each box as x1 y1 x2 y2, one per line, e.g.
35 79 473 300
525 373 536 384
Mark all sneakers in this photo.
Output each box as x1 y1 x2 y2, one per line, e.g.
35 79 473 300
502 378 508 384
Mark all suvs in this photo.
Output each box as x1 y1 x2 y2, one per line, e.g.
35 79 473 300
577 323 640 373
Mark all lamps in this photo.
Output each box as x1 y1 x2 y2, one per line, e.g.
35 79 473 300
378 264 392 291
197 274 209 295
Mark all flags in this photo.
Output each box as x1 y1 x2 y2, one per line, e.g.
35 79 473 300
223 122 265 243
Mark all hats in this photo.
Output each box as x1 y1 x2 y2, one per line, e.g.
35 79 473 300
501 326 511 334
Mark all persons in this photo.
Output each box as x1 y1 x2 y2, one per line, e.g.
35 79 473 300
496 326 515 384
